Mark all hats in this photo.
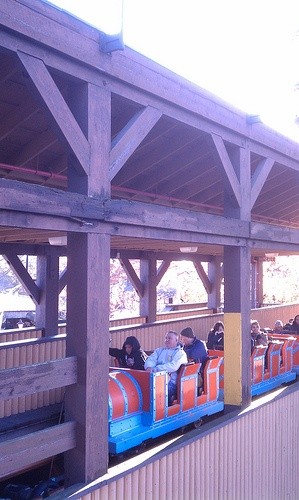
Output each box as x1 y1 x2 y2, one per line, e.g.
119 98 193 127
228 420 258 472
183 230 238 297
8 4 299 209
181 327 194 338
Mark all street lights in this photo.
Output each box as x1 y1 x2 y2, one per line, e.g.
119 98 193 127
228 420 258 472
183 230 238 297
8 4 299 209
14 318 27 332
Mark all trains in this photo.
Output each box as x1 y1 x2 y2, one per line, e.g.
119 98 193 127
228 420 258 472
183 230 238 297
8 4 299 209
85 326 299 457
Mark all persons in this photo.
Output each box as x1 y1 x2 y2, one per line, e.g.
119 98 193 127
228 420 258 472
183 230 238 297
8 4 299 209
109 336 148 370
176 315 299 396
144 331 188 406
